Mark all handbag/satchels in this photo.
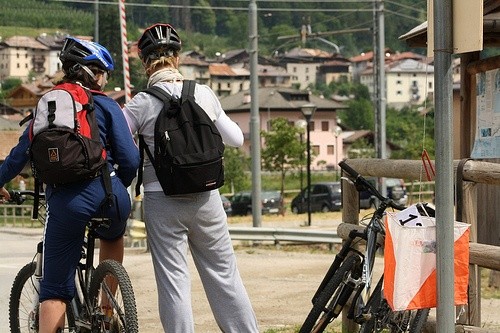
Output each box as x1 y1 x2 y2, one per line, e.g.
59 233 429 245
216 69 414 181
385 201 472 312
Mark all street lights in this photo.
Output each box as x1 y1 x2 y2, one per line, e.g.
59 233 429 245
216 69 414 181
293 119 307 191
300 103 317 226
331 127 341 182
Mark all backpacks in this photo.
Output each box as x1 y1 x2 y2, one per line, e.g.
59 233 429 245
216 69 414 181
134 79 226 196
25 82 114 186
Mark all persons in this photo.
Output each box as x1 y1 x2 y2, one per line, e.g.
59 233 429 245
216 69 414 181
0 38 141 333
122 23 259 333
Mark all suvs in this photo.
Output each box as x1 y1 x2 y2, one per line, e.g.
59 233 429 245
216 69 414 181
290 176 407 214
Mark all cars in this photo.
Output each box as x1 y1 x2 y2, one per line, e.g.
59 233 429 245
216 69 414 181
219 192 282 216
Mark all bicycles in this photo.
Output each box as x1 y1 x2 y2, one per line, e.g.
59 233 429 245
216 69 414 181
0 190 138 333
299 161 435 333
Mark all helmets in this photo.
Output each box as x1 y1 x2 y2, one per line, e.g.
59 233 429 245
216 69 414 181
58 36 115 75
138 22 182 60
